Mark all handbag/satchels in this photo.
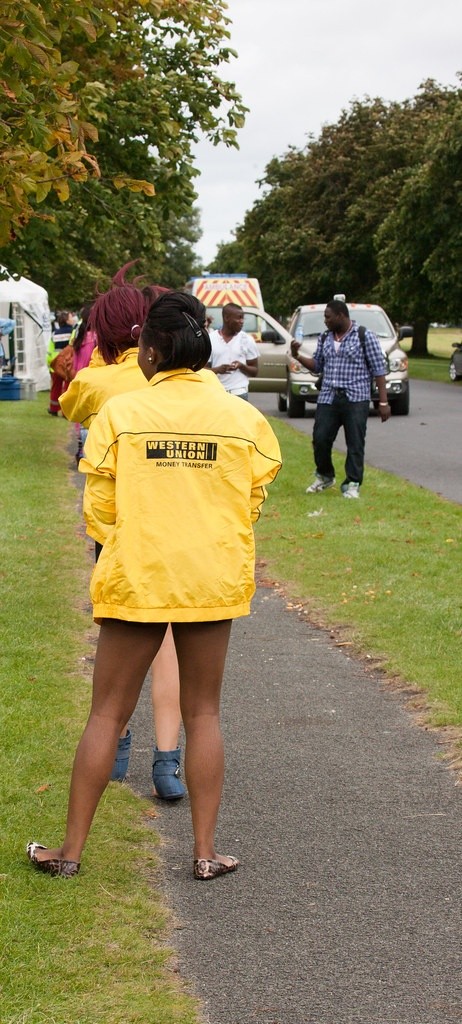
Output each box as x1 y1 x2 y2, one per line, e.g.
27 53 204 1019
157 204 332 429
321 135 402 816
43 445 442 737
51 345 73 380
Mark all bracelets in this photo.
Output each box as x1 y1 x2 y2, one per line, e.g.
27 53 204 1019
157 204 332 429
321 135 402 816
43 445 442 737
296 353 301 360
378 402 389 407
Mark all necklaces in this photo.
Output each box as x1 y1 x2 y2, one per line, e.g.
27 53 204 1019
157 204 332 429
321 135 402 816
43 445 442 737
336 322 352 341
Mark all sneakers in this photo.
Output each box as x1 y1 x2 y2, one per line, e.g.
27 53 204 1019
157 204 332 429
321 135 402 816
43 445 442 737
342 482 359 500
306 474 336 493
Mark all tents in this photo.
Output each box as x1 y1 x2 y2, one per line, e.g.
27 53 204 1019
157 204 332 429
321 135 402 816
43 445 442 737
0 265 52 394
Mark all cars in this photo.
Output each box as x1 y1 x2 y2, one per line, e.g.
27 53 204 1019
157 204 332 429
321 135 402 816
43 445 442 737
240 295 415 418
448 340 462 381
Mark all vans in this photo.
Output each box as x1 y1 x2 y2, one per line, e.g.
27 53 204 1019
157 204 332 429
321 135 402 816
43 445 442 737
184 272 266 343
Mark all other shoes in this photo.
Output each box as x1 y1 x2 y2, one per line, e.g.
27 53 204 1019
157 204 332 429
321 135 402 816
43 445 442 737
193 855 238 879
26 842 81 879
48 407 58 416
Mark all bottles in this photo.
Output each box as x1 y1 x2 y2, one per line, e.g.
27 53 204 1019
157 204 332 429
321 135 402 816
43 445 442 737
294 323 304 345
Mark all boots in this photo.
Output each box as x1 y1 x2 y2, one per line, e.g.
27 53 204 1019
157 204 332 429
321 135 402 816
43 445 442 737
109 729 131 782
153 745 186 800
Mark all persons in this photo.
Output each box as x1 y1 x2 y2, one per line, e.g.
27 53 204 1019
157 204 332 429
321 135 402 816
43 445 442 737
0 319 15 377
28 283 282 881
291 300 391 500
